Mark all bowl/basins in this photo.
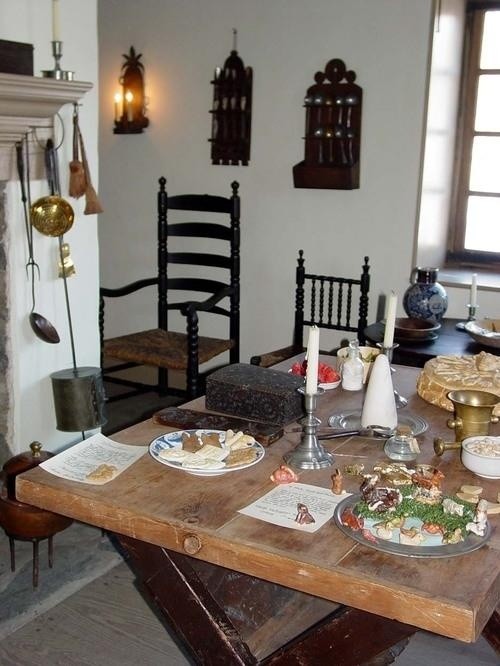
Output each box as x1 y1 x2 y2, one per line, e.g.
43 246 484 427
378 316 443 338
464 318 499 347
461 436 500 481
335 345 379 385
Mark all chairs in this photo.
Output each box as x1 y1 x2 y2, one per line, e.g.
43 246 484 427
99 176 240 405
251 249 370 367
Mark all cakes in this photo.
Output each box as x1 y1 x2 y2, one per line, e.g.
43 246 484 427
416 351 500 417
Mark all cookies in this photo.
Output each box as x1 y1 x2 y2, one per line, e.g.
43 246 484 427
159 428 258 470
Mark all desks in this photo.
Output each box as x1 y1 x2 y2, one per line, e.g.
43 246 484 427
364 317 500 357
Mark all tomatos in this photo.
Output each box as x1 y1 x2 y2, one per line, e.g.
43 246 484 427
290 360 340 383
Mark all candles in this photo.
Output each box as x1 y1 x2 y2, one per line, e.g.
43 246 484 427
383 290 397 347
114 94 119 120
304 324 320 393
127 90 134 120
470 274 477 305
53 1 61 40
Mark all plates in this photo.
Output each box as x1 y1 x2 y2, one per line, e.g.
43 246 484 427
147 429 267 478
331 493 492 559
287 367 341 390
378 331 439 343
329 409 431 442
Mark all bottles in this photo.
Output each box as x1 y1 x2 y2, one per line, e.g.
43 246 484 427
341 338 365 392
383 425 421 462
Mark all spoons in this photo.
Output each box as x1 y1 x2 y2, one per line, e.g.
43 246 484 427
304 91 358 165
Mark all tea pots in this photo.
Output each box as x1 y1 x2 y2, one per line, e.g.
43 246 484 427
402 265 449 323
